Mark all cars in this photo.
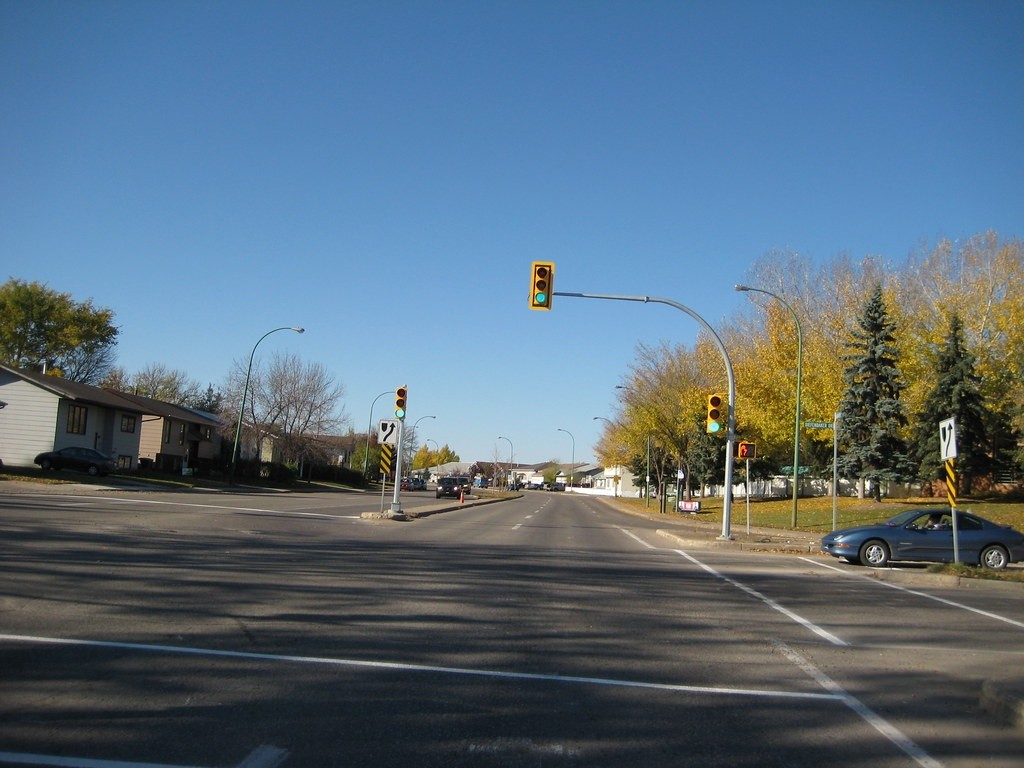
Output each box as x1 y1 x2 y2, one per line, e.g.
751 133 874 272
473 474 565 492
399 477 427 491
820 506 1024 570
434 476 461 498
34 447 119 475
457 477 472 495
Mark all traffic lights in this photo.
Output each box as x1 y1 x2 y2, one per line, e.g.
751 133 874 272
529 261 554 309
739 440 757 462
708 393 722 436
395 386 407 420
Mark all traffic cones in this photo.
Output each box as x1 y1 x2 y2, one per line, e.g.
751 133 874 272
460 490 465 504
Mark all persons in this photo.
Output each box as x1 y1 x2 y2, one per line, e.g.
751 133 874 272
913 514 944 529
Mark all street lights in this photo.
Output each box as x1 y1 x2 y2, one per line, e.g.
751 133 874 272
402 414 439 474
497 435 514 472
734 282 803 529
558 428 575 486
229 326 307 463
